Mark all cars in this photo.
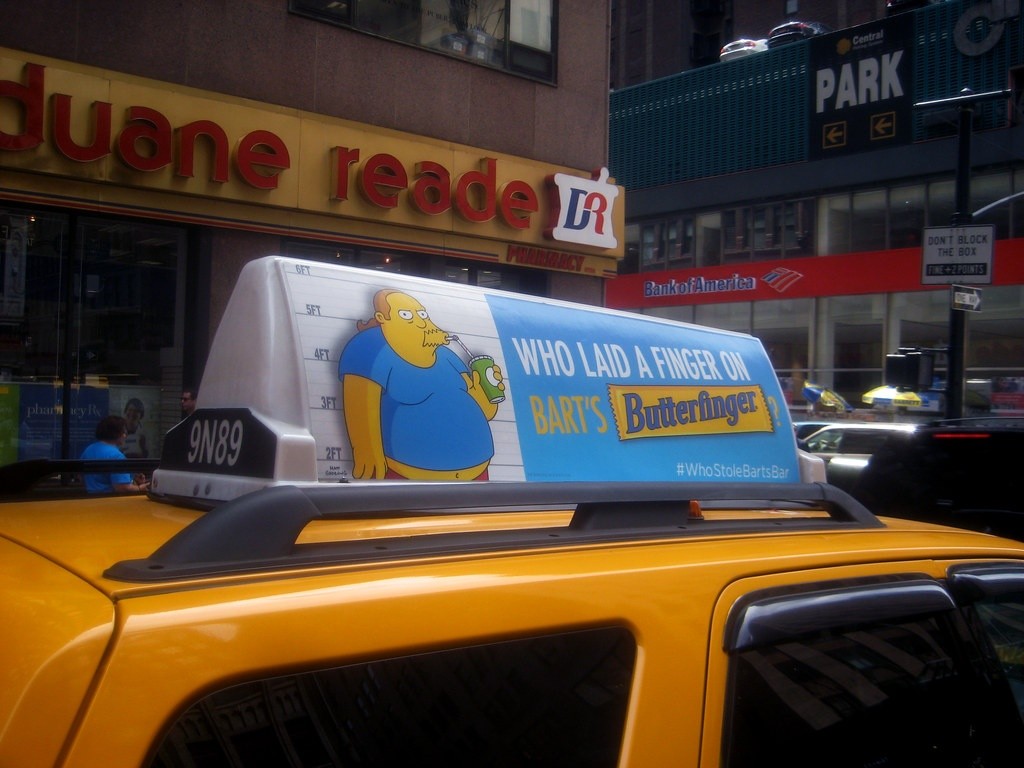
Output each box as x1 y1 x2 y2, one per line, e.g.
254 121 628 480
719 20 838 64
0 254 1024 768
